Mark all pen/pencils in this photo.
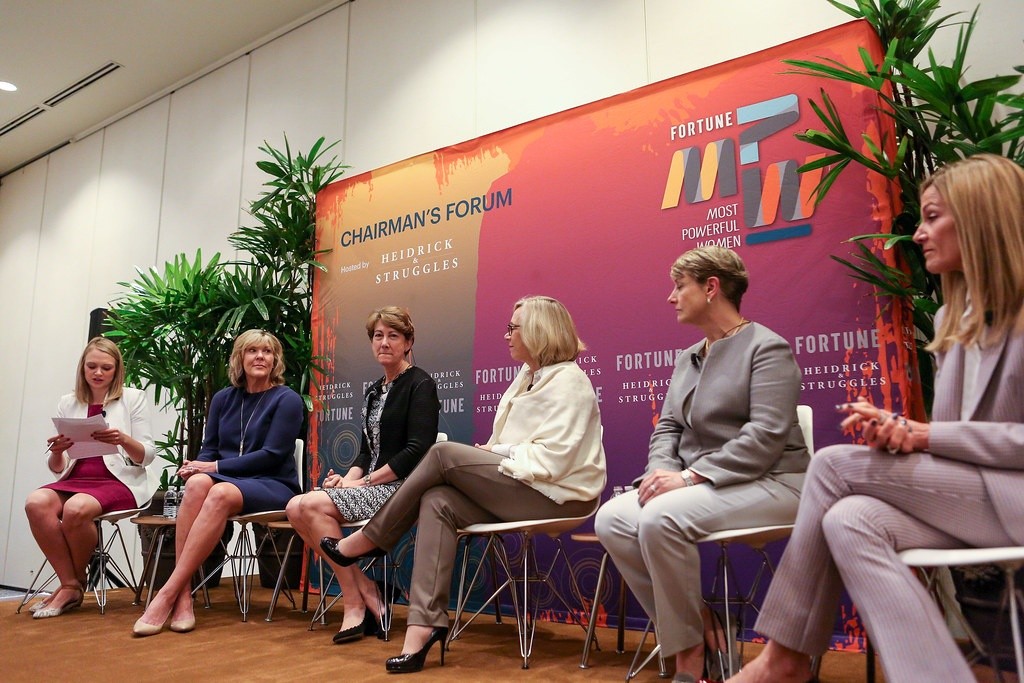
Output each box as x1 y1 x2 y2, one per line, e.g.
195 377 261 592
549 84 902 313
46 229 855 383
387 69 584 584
44 442 55 454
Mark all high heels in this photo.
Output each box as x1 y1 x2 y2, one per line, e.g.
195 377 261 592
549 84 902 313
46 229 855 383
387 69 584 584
170 599 195 632
133 602 174 635
374 580 402 639
385 626 448 671
320 536 397 567
334 605 376 643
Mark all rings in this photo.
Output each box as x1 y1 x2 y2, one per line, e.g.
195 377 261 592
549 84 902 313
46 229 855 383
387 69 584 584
650 484 656 492
189 467 192 470
887 443 899 454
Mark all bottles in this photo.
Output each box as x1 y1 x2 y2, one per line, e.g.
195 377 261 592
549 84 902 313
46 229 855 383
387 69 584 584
163 486 186 518
609 486 634 500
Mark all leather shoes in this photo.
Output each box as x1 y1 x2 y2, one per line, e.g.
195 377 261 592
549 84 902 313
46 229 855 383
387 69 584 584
28 598 49 612
672 641 712 683
707 607 739 683
33 584 85 618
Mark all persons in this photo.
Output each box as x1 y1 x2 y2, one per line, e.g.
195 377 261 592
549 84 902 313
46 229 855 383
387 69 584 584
22 337 156 618
284 305 444 642
130 330 305 638
595 247 811 683
321 293 608 673
721 153 1023 683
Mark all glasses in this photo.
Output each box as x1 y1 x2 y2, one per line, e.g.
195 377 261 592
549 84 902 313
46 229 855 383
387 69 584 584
507 325 520 336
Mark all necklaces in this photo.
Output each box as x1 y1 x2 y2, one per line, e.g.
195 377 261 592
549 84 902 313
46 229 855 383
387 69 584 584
238 391 265 457
386 363 411 381
706 317 743 353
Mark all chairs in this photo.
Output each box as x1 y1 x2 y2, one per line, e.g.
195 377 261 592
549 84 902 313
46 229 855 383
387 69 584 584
446 423 604 671
15 496 153 614
627 404 815 683
310 432 449 640
190 438 306 621
862 545 1024 683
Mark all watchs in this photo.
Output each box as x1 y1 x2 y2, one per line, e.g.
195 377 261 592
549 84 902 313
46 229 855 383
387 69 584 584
680 469 695 487
364 475 371 486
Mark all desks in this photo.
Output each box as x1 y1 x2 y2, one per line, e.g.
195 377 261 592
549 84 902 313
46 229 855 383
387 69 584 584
131 516 212 609
570 533 627 669
264 519 329 625
453 528 503 637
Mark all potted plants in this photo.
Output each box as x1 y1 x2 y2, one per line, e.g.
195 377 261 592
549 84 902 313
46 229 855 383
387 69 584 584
101 130 362 596
